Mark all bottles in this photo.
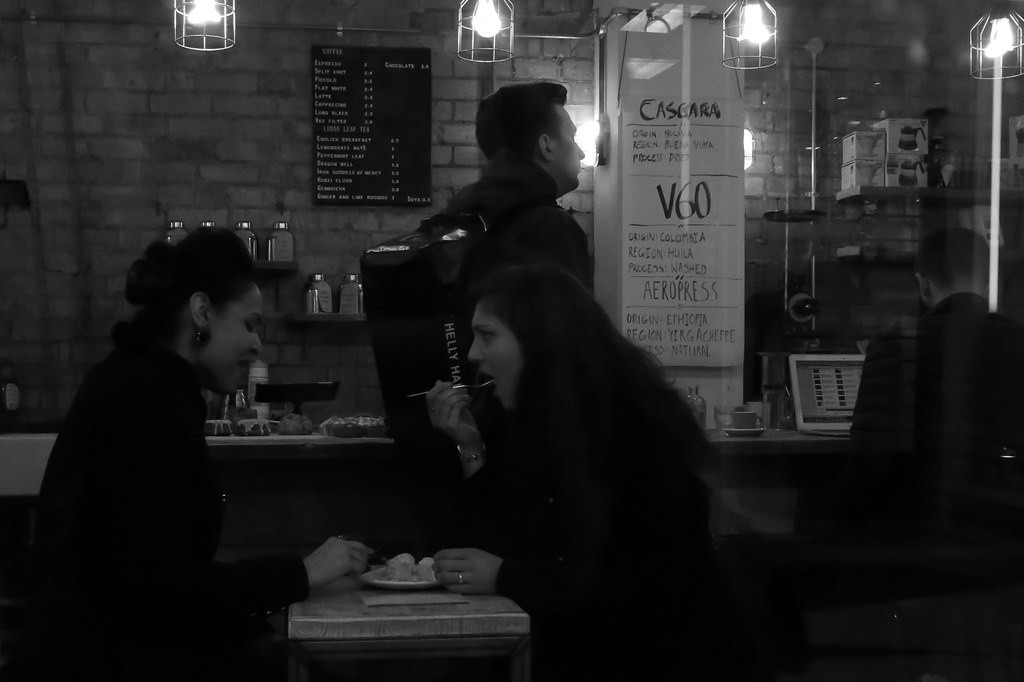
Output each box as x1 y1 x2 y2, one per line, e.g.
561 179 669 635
686 383 707 435
246 358 271 419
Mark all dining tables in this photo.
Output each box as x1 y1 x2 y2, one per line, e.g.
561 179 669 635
287 575 533 682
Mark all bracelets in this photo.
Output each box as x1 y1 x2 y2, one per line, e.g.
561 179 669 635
456 444 486 464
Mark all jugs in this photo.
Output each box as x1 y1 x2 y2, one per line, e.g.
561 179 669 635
756 349 791 431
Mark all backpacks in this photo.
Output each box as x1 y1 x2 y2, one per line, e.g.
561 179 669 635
361 196 557 549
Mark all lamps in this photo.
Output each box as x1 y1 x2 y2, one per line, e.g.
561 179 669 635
456 1 515 62
172 1 240 51
722 0 779 69
968 1 1024 80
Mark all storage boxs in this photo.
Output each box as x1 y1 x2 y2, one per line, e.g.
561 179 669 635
839 118 930 189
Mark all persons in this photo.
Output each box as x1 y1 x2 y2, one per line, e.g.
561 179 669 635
425 264 757 682
408 87 594 559
847 228 1024 594
21 227 376 682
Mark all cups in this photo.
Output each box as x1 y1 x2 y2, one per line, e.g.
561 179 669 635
731 411 757 428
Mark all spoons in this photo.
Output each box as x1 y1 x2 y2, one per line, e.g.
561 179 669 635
408 377 495 401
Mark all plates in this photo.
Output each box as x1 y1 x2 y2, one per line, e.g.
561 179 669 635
357 567 441 591
724 427 765 436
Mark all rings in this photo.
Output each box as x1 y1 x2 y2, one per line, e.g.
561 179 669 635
459 571 464 584
